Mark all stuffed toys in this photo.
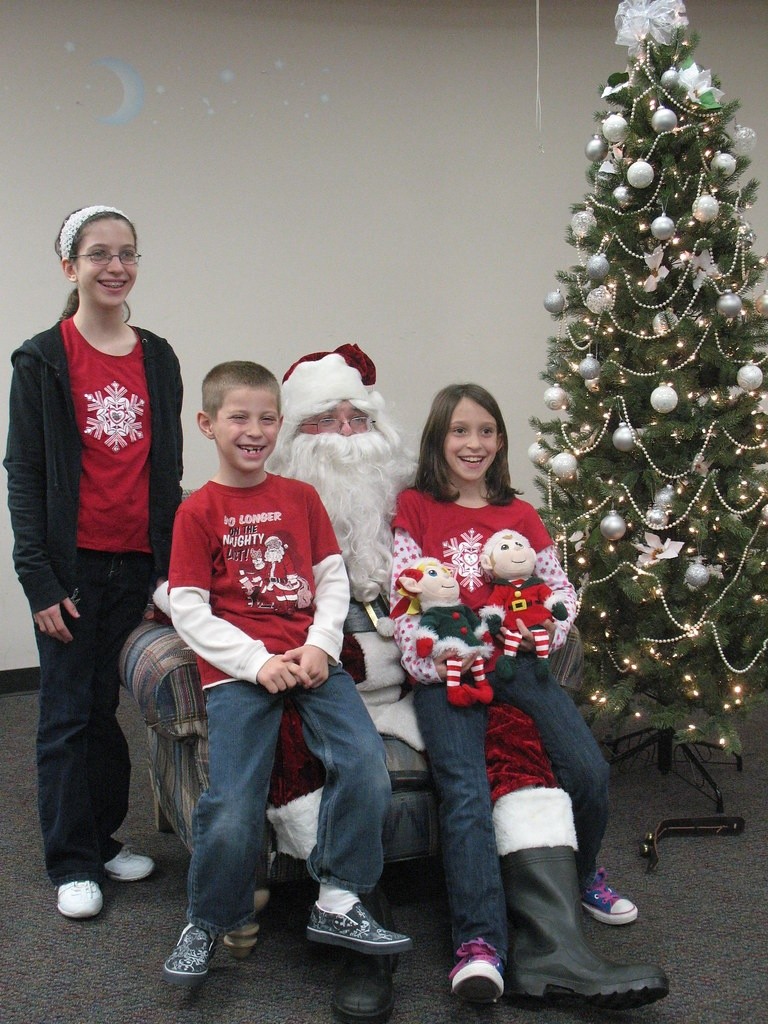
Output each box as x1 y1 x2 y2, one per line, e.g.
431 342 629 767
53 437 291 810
481 529 567 676
396 556 493 703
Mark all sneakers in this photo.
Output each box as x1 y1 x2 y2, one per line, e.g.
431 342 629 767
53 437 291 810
57 878 103 917
162 924 219 984
449 938 504 1003
104 848 154 881
306 901 413 954
580 867 638 925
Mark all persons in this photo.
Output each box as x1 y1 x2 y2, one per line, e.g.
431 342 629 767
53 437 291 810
149 343 668 1021
391 384 639 999
3 204 183 922
166 362 412 978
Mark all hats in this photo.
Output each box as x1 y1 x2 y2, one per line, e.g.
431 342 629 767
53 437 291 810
282 342 376 408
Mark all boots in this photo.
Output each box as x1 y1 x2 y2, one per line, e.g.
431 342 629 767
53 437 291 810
491 786 669 1009
266 786 400 1024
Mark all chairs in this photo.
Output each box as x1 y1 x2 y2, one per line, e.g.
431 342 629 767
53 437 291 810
115 492 582 920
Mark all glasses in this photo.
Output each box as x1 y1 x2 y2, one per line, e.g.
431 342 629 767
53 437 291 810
298 416 376 434
69 250 142 265
